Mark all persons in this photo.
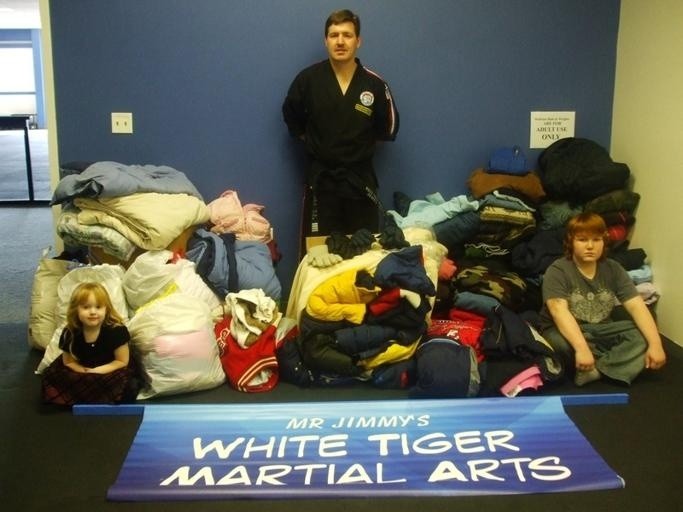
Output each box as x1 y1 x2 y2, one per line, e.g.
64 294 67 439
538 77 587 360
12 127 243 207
37 281 143 417
280 9 399 259
538 212 666 390
